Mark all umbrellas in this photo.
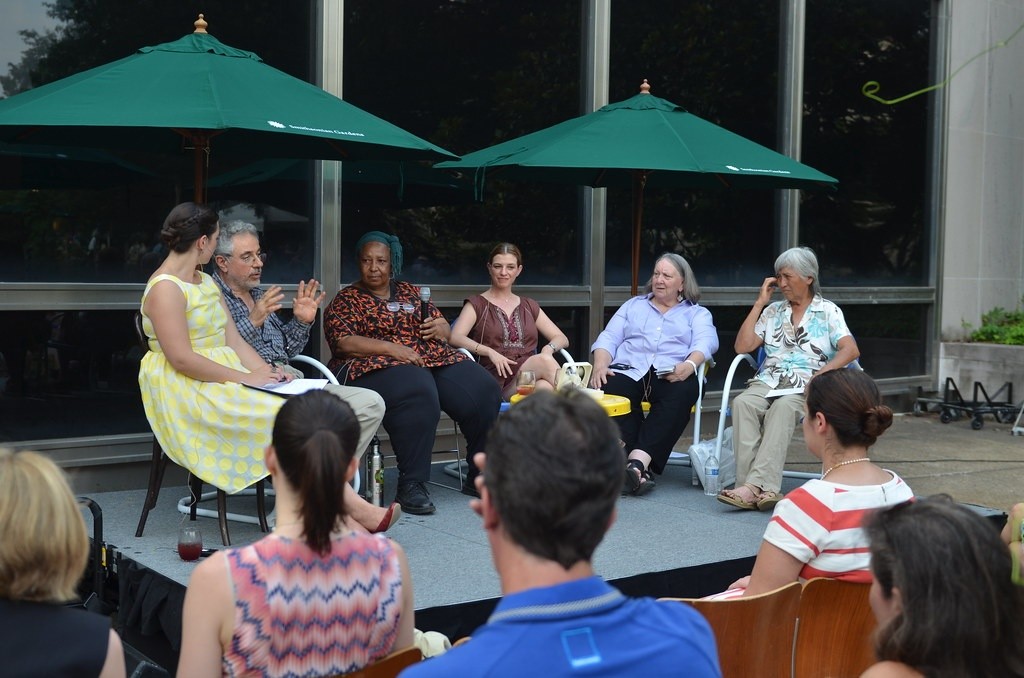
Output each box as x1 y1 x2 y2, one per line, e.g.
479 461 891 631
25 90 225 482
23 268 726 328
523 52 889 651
0 12 463 210
430 79 842 294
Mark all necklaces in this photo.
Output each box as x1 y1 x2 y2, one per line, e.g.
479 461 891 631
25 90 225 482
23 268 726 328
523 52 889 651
820 458 871 480
491 290 513 303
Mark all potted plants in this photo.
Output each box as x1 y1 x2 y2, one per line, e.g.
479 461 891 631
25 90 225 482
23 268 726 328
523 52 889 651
944 291 1024 420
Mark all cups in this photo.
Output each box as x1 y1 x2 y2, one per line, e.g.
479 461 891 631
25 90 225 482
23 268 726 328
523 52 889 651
516 370 536 396
555 368 572 390
178 528 203 562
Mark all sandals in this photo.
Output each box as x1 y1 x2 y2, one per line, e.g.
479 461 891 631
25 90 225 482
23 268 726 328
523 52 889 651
717 484 759 509
624 458 645 496
633 469 655 496
757 491 783 511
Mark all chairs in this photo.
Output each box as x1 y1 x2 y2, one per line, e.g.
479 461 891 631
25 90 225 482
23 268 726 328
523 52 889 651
442 317 579 479
793 577 881 678
178 355 362 530
425 419 463 492
639 361 709 486
655 582 801 678
134 312 270 547
712 332 865 494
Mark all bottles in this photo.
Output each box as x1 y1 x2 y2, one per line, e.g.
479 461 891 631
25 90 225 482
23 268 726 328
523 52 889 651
365 436 384 508
704 450 719 496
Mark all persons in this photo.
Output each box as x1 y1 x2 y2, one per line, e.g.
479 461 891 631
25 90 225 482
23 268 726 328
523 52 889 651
1 447 127 678
175 388 414 678
730 368 917 603
398 385 723 677
1000 501 1024 585
322 230 501 515
450 242 627 449
213 217 387 486
138 201 402 536
855 492 1024 678
717 246 861 511
588 253 719 494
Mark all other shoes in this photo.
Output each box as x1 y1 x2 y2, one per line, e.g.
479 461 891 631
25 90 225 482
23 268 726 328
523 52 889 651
367 503 402 534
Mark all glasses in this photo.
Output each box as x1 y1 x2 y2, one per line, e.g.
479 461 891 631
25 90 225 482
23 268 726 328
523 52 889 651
386 302 415 313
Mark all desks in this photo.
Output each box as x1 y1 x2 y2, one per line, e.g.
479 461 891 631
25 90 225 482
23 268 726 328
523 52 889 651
510 391 631 418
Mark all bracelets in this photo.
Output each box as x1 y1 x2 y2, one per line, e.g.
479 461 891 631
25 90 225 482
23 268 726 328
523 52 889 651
548 343 555 352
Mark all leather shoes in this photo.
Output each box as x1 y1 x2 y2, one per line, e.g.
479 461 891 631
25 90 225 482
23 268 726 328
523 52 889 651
395 481 436 514
462 473 481 498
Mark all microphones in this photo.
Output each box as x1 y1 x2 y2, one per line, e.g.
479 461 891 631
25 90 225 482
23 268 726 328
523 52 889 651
420 287 431 341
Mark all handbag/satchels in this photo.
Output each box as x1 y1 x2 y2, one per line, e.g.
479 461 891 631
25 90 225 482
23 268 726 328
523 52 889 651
687 425 737 488
